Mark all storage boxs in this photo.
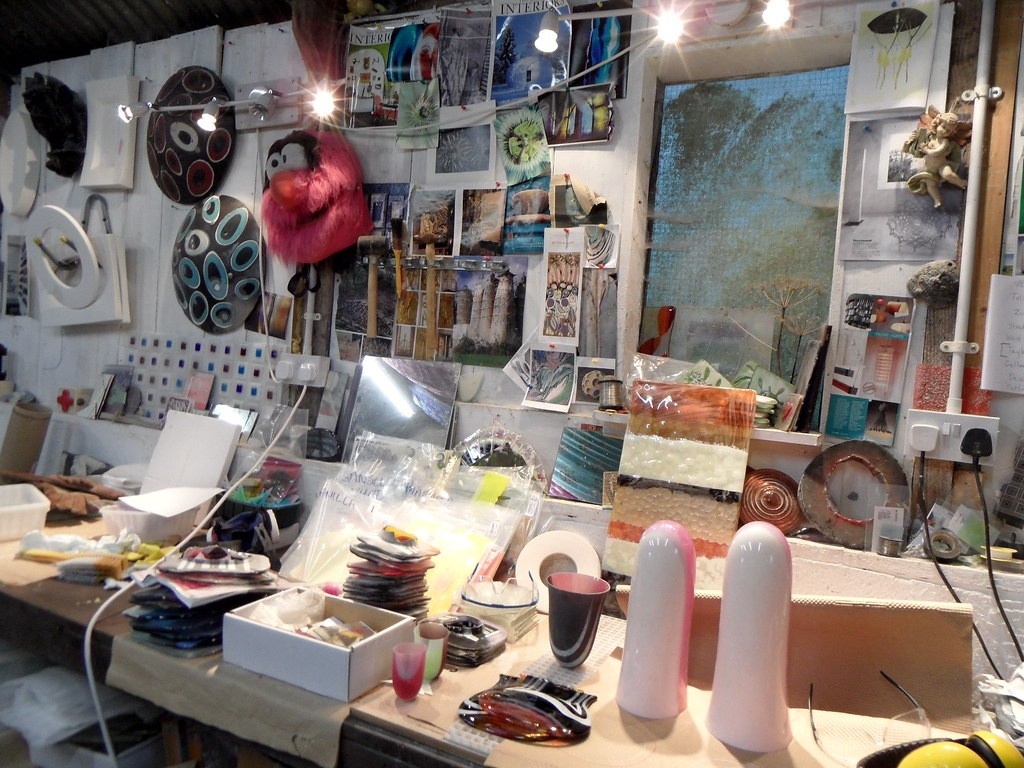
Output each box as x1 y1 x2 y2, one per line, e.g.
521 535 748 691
0 483 204 548
222 588 417 702
27 731 164 768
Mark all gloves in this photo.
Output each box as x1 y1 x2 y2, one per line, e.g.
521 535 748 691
43 485 100 515
49 475 127 500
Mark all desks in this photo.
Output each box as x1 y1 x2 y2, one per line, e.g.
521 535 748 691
0 512 1024 768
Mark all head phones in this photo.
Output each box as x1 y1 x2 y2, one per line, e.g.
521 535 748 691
898 731 1024 768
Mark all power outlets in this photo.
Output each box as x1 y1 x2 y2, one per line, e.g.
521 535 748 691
904 409 1001 467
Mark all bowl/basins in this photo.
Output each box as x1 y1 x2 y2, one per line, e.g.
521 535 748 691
0 483 51 542
99 503 199 545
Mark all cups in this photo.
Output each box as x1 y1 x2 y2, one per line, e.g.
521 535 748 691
393 622 450 701
547 571 611 668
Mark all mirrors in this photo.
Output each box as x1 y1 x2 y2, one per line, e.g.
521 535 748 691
341 355 462 469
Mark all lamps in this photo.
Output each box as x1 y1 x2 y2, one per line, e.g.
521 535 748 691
114 7 565 130
